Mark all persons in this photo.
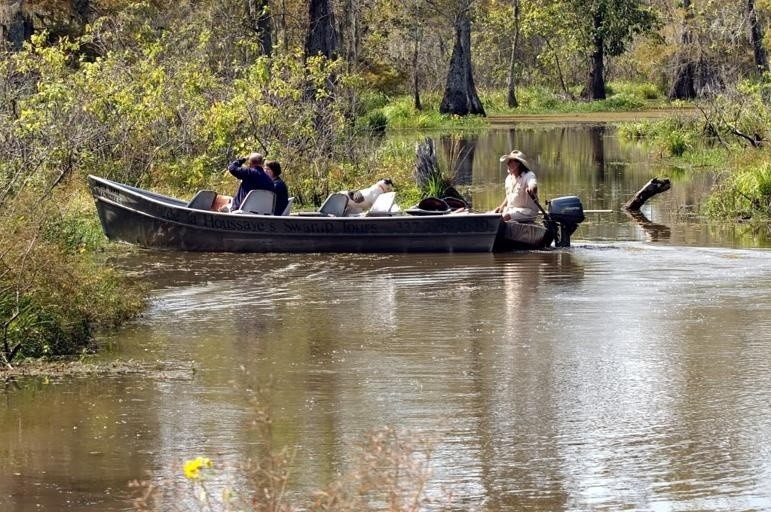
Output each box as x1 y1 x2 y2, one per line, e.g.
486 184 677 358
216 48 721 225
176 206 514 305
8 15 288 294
218 150 273 213
488 149 540 222
263 160 289 215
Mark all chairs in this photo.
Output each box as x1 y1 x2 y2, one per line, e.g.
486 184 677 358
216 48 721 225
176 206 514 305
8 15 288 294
187 189 403 218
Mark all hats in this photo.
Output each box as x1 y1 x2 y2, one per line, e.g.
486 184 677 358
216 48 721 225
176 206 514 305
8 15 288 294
499 151 531 171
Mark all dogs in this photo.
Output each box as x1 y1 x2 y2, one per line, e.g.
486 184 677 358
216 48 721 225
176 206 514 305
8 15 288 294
336 177 394 217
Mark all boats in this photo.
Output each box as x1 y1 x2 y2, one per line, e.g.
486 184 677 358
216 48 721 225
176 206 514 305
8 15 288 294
86 168 585 257
